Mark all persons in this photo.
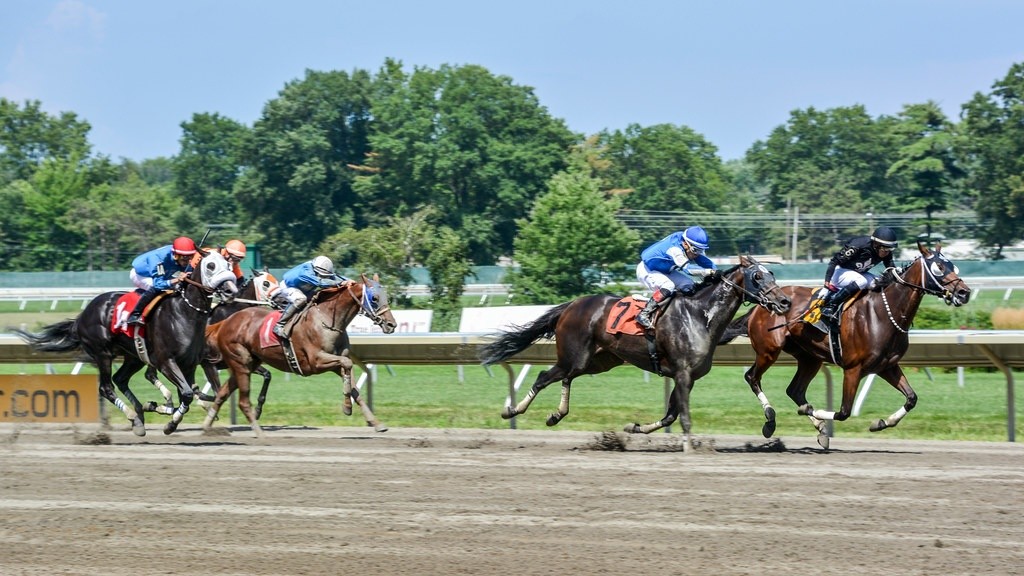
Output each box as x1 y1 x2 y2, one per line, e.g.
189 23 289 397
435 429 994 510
818 226 900 321
126 236 197 326
271 255 356 339
635 227 721 330
179 238 250 299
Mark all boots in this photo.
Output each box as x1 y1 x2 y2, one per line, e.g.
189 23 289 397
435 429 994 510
634 287 672 329
127 287 160 326
821 282 861 321
273 303 299 339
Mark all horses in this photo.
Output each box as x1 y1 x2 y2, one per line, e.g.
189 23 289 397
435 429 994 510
715 239 971 449
141 266 289 420
476 254 792 454
205 272 397 446
8 243 238 437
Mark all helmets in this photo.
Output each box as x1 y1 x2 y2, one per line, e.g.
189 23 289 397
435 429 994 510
225 240 246 257
173 237 196 254
313 255 335 276
682 226 710 249
871 226 898 249
